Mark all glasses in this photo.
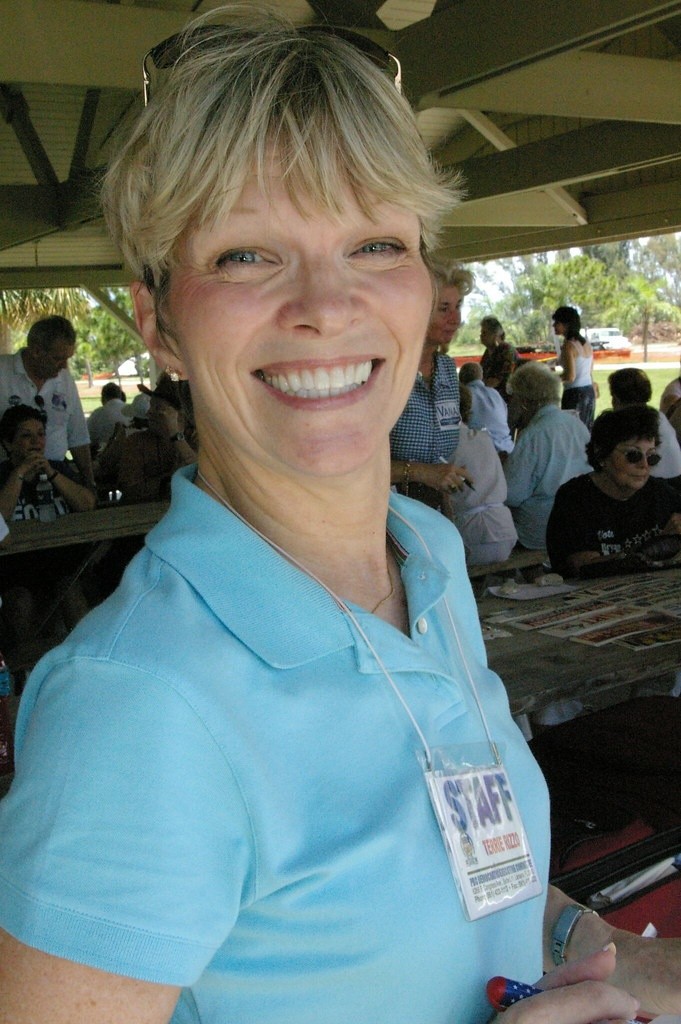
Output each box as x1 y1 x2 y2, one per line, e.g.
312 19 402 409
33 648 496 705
35 394 49 425
615 449 662 466
140 24 403 112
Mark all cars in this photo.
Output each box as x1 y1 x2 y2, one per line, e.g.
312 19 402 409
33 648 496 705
115 349 156 378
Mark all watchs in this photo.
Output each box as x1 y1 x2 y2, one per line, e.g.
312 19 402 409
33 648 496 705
170 431 185 443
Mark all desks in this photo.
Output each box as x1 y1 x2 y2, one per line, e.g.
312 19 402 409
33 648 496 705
473 567 681 736
0 502 172 639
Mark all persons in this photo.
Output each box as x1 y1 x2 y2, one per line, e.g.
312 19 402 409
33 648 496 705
97 394 152 472
87 382 134 482
459 356 516 466
0 2 681 1024
504 362 596 556
436 386 520 564
609 369 680 515
545 407 681 577
479 316 522 388
0 314 95 509
0 404 96 698
551 305 595 433
95 373 198 506
390 253 475 521
660 377 680 449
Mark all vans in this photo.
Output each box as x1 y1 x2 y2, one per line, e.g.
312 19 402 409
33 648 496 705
579 327 632 351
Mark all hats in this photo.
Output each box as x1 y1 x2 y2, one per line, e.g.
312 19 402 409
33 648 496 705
138 381 181 411
121 393 150 419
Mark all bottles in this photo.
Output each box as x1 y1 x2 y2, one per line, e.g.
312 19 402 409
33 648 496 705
36 475 56 523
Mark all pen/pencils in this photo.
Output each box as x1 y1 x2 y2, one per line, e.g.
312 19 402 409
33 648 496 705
486 974 659 1024
439 455 475 491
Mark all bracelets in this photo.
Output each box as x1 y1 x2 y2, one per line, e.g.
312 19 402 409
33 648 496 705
9 470 23 480
403 457 418 483
550 904 599 967
47 470 59 482
